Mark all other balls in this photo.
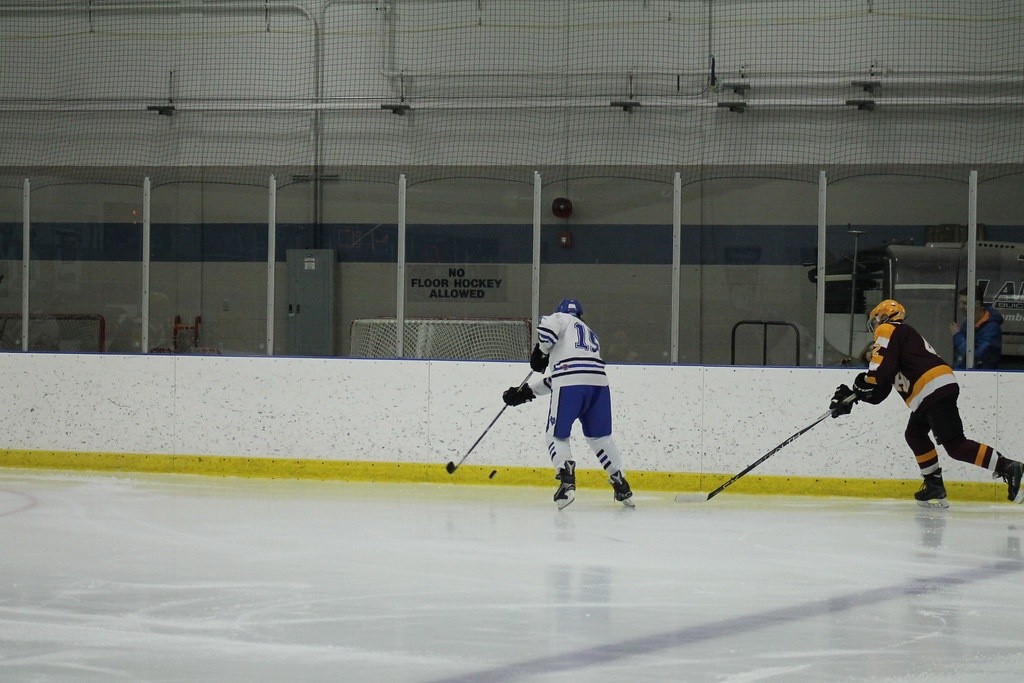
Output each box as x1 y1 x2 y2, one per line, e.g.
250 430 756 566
488 469 497 479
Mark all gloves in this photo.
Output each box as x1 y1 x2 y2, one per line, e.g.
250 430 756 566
829 384 858 418
503 382 536 407
530 343 549 374
853 372 877 402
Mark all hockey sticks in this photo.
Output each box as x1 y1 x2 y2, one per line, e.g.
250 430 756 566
674 393 857 504
445 368 534 474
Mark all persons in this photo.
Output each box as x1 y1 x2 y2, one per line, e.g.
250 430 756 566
502 299 634 510
829 299 1024 508
950 285 1004 371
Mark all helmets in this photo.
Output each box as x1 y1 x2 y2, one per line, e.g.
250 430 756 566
556 298 585 318
867 300 906 335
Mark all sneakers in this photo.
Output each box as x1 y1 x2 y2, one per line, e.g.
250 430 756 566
992 451 1024 504
553 460 576 510
914 467 949 508
609 470 636 507
